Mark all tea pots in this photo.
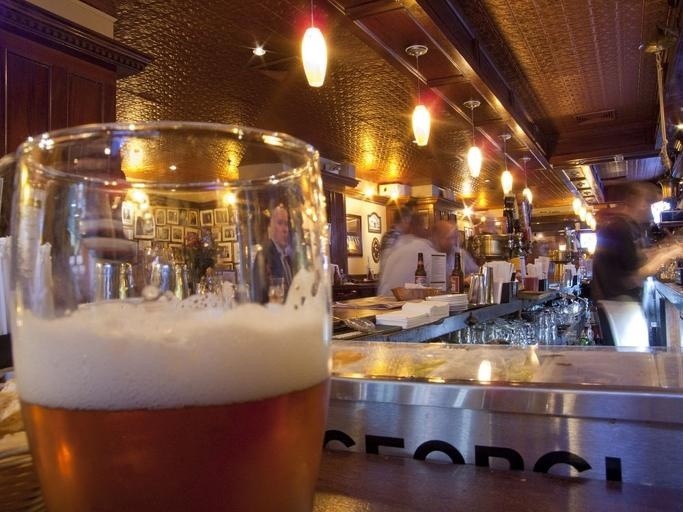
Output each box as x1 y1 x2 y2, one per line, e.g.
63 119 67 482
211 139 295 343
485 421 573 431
658 174 683 208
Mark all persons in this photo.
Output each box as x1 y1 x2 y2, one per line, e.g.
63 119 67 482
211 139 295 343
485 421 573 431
185 226 216 297
373 187 481 306
588 179 683 345
251 204 299 307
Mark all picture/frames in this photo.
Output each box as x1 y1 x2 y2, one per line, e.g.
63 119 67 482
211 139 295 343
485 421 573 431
367 236 381 274
345 213 361 235
365 210 382 233
417 207 473 243
345 235 362 258
118 200 249 285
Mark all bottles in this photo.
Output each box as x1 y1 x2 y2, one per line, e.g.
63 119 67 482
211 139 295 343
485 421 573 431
413 252 428 287
449 251 466 295
469 259 587 306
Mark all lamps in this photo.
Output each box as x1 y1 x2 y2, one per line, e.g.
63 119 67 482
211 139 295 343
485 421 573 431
298 1 329 91
402 41 436 147
463 98 484 179
496 131 514 194
520 155 532 205
571 194 597 232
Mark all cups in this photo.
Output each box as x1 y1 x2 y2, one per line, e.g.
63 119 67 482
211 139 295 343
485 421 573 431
453 306 561 345
8 121 335 512
267 274 286 303
